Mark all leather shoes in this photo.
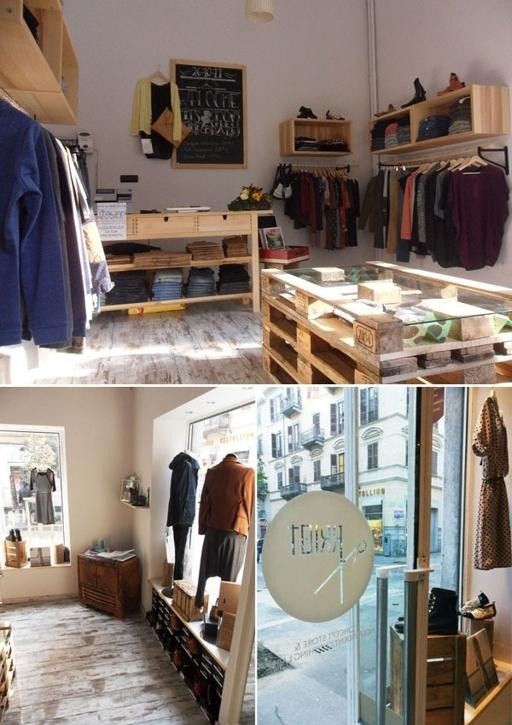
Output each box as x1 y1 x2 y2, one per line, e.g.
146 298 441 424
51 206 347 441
297 106 317 119
327 110 345 120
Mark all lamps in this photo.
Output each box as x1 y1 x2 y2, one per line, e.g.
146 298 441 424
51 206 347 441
246 0 274 23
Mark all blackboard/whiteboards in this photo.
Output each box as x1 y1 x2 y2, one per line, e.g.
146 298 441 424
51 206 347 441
170 59 249 169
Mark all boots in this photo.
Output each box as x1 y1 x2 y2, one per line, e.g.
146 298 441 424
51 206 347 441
402 77 427 108
394 588 458 634
5 529 16 542
14 528 22 542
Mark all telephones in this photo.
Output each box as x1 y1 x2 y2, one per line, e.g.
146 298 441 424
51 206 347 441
94 538 109 553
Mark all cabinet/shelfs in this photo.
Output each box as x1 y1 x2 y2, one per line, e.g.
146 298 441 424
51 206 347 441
279 117 354 157
1 0 81 126
76 551 141 619
260 258 512 384
148 577 229 724
368 84 507 153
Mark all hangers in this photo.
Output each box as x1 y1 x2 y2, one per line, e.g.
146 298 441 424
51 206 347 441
377 145 491 177
294 161 358 184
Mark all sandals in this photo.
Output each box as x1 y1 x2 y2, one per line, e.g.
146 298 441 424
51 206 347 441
438 72 465 95
374 105 396 117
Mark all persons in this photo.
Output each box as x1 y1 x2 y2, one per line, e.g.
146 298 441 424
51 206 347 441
198 452 252 622
18 482 35 521
168 447 200 591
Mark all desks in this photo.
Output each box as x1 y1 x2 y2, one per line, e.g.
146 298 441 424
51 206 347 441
91 209 273 315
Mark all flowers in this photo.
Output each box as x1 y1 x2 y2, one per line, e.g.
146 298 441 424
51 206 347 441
227 182 274 210
